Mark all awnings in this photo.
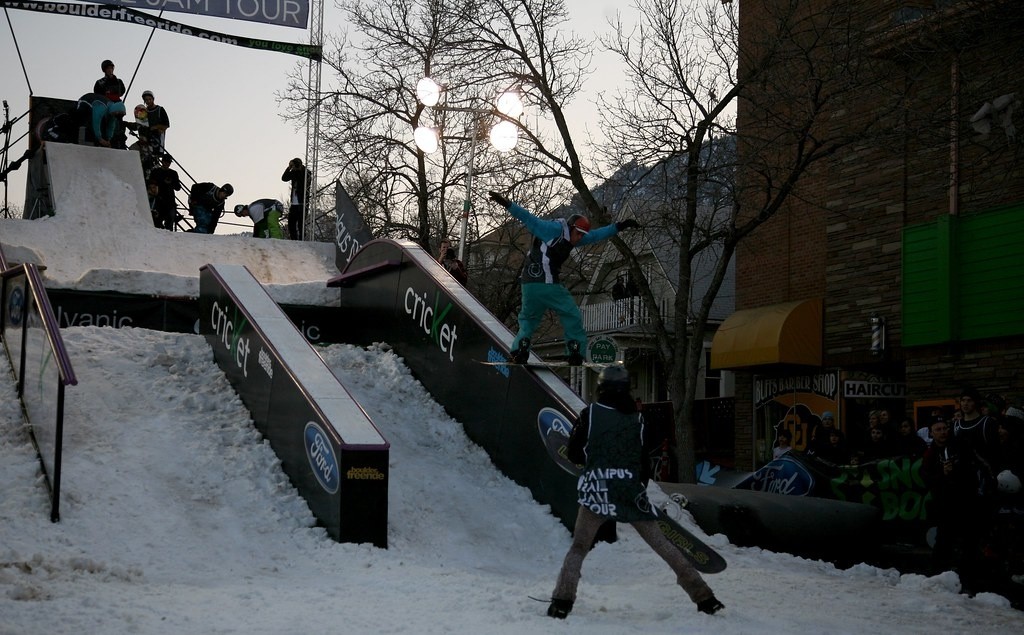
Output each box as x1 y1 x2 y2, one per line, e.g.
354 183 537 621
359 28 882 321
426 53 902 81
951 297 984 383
710 296 824 367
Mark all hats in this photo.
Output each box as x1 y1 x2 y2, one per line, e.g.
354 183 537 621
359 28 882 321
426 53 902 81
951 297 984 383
961 384 980 402
928 416 946 438
820 411 833 422
142 91 152 99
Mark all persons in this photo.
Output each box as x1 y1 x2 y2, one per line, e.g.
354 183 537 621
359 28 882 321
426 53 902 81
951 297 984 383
185 182 233 234
94 60 127 152
282 158 311 241
767 389 1024 591
129 93 181 232
77 93 126 148
436 241 465 283
234 199 283 239
489 190 645 366
546 364 724 619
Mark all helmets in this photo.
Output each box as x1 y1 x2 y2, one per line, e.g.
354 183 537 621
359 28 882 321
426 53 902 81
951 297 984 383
220 183 234 195
567 214 590 234
234 205 244 217
597 366 631 387
102 60 114 72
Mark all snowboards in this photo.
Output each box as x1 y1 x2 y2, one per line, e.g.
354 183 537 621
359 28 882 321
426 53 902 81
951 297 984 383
479 359 626 369
536 406 728 575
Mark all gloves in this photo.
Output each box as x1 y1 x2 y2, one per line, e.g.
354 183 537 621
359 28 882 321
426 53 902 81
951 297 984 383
616 219 640 231
489 191 509 207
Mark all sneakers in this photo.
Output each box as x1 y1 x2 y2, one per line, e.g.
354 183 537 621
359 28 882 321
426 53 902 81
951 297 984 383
509 338 529 364
567 340 584 365
697 596 724 615
548 599 573 619
